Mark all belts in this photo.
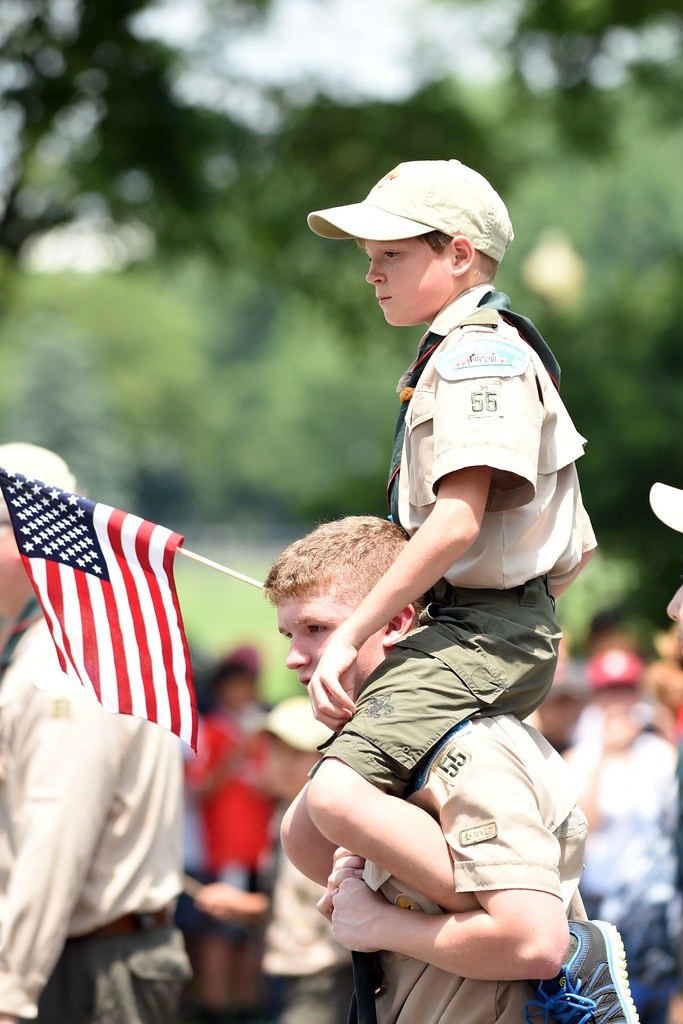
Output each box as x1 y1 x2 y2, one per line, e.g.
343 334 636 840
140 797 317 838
72 909 172 942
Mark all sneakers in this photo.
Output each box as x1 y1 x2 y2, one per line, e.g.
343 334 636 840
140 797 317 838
525 920 642 1024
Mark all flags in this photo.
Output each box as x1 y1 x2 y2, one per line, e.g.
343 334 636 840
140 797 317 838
0 469 198 754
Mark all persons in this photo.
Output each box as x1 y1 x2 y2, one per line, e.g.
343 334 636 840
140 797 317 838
176 482 683 1024
0 443 193 1024
264 516 588 1024
278 160 640 1024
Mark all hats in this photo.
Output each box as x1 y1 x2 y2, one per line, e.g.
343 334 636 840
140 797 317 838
2 441 79 501
308 156 514 266
649 482 683 536
588 647 642 693
211 647 258 687
